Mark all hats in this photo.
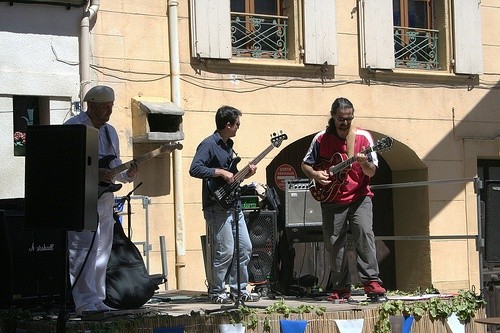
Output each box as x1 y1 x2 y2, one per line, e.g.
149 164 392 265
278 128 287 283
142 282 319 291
83 85 115 103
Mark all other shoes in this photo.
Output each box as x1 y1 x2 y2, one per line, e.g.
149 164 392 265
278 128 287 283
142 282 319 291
233 292 259 302
210 293 232 304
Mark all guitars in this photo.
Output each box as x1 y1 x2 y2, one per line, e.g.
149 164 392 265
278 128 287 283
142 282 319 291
308 136 393 204
206 130 289 209
97 142 183 199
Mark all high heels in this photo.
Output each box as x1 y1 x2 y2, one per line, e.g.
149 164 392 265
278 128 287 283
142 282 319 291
95 302 118 311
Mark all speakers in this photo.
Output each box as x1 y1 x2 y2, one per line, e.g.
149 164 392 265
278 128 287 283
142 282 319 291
283 179 322 228
26 124 99 230
0 198 72 308
241 208 278 284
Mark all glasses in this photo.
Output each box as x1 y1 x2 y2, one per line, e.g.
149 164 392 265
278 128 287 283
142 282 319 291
335 116 353 121
236 123 240 126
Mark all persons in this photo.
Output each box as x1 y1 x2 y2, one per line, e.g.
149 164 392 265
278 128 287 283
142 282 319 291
190 106 260 304
301 96 386 301
64 85 141 317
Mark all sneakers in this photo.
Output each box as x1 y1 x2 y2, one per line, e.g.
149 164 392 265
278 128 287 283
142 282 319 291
364 281 386 293
327 290 351 301
